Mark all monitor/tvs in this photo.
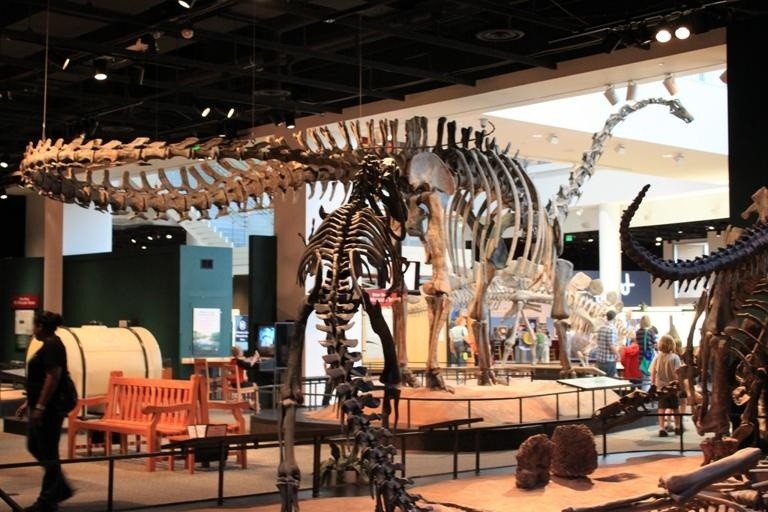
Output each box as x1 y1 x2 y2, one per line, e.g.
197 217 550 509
258 324 276 352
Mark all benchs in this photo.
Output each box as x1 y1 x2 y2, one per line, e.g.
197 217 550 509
156 358 260 470
65 370 198 474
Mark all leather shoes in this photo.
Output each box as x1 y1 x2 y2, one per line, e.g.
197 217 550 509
24 501 59 511
675 428 681 434
660 430 667 436
38 484 72 502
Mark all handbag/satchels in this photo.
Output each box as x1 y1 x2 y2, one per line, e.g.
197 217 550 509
638 355 651 376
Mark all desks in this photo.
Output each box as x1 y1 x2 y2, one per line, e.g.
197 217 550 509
170 427 248 473
180 356 271 384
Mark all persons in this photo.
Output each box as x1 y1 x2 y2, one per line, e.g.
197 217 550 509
15 309 79 512
595 310 703 438
229 345 262 411
448 316 469 366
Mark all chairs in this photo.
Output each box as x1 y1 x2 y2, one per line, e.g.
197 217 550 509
192 357 221 400
220 363 261 415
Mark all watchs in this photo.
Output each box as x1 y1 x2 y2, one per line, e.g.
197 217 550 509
33 402 47 412
